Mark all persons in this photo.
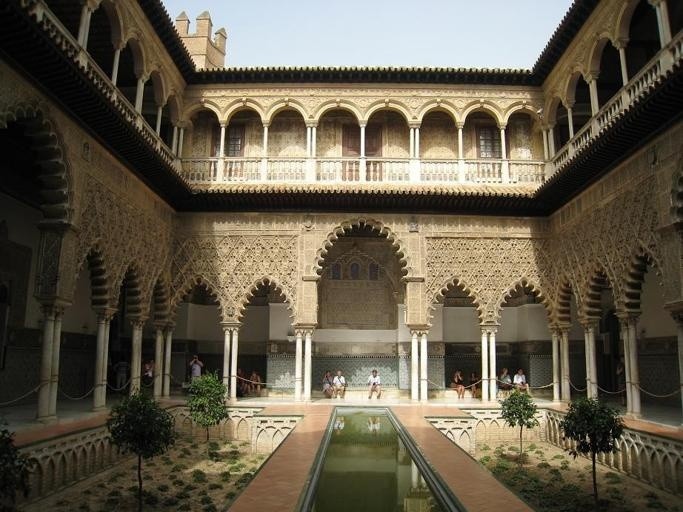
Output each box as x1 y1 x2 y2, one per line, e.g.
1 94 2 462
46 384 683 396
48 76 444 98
142 359 154 386
452 369 465 400
333 415 345 434
498 367 513 390
322 370 334 399
512 367 530 395
469 372 480 399
249 371 261 396
112 360 130 392
330 369 346 397
236 367 248 395
368 416 381 436
614 354 627 406
189 355 204 379
366 369 381 399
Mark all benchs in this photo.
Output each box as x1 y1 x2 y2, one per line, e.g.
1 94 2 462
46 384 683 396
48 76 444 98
240 387 530 401
